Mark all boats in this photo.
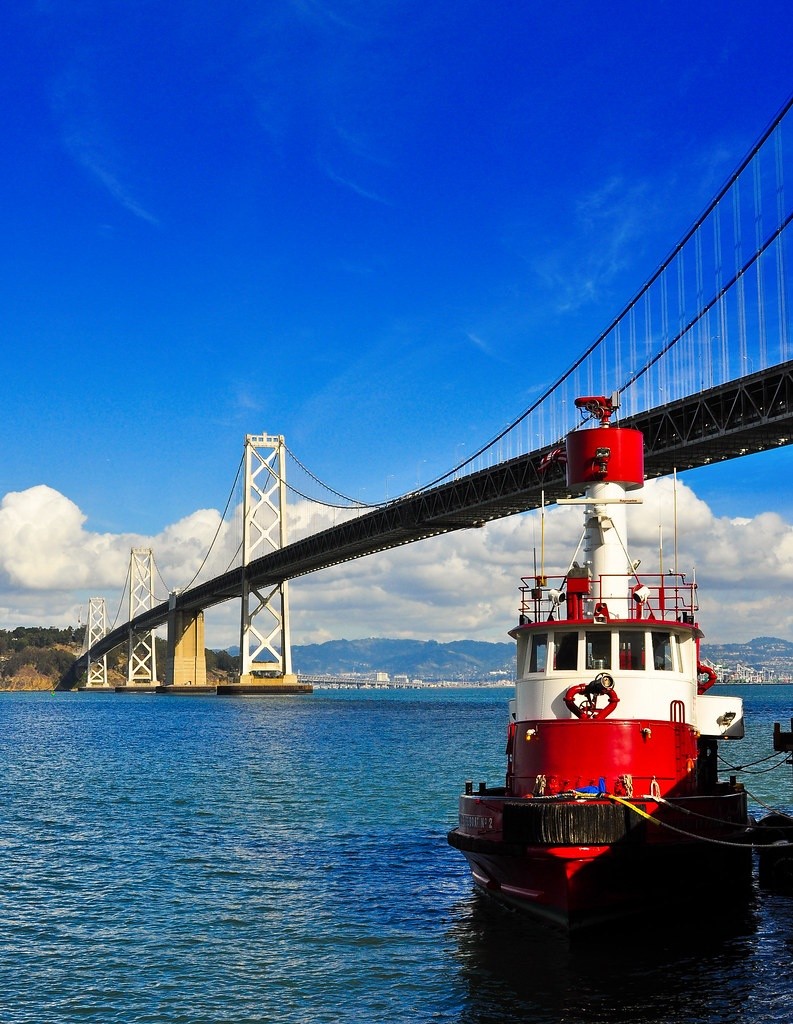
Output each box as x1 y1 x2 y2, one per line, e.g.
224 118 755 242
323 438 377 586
445 390 753 929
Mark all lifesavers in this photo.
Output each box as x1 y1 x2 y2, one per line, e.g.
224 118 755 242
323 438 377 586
506 723 515 754
